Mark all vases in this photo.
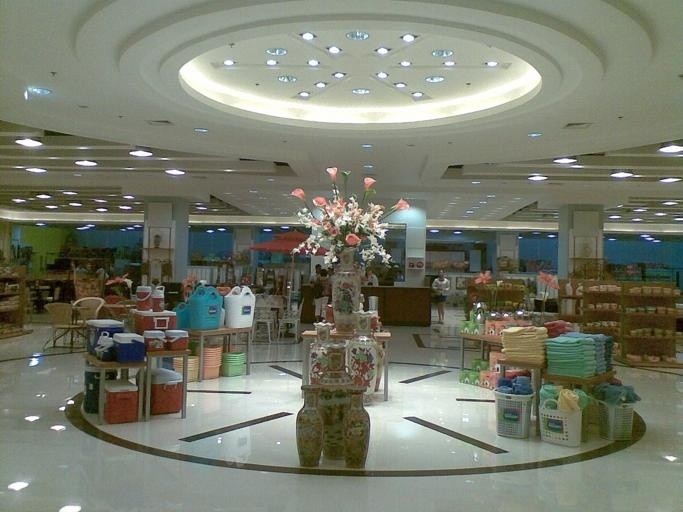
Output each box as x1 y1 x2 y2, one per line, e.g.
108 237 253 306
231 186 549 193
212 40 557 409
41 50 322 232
295 311 391 469
331 248 362 334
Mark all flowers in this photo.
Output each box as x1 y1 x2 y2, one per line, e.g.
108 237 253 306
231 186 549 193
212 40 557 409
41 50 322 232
106 273 134 332
291 167 410 279
474 270 493 285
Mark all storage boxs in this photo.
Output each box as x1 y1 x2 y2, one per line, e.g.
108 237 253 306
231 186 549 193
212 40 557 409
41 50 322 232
83 286 192 423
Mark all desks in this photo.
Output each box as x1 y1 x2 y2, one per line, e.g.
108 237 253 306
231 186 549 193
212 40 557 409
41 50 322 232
496 359 617 442
188 327 251 383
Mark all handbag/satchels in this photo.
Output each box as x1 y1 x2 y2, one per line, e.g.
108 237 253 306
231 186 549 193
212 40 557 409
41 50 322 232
312 276 324 298
173 284 256 331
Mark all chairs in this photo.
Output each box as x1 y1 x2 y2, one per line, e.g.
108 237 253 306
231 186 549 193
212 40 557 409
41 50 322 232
42 297 106 352
251 297 305 343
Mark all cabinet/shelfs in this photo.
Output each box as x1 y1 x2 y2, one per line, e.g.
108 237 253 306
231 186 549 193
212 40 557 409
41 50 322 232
460 278 683 369
0 265 33 341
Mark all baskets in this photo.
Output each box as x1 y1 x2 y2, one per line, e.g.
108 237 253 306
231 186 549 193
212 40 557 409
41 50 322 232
491 383 536 439
538 392 636 447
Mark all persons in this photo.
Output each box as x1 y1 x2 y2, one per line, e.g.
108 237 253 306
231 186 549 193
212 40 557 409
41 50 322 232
328 263 343 286
390 269 403 282
361 266 379 287
312 268 330 322
430 268 450 325
307 263 321 284
